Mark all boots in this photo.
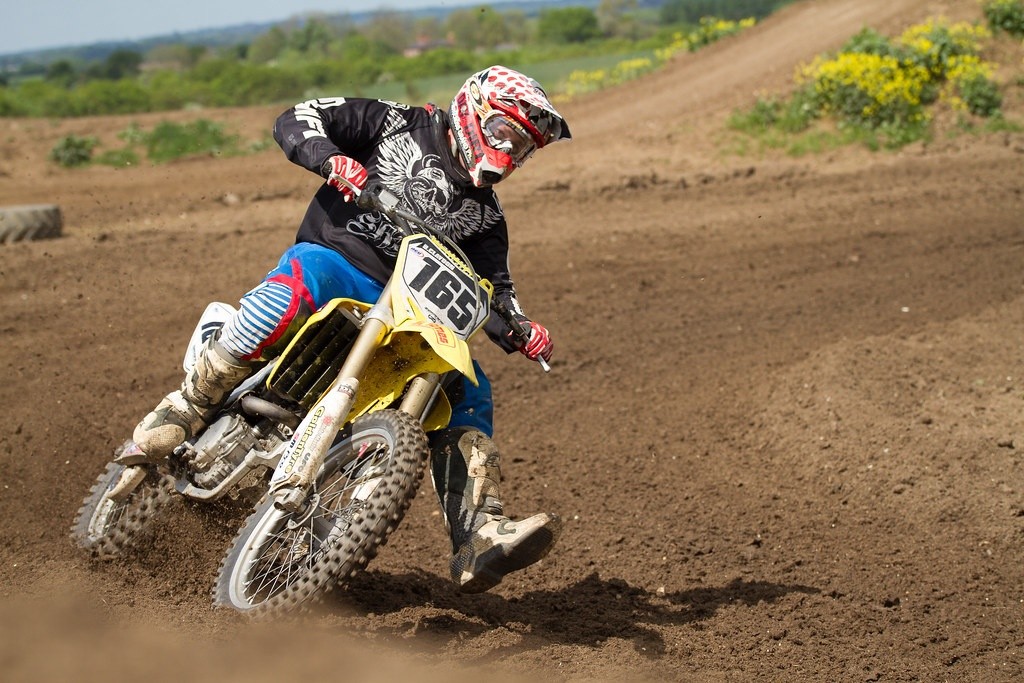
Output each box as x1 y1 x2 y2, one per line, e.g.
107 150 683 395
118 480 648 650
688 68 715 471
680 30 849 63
134 325 252 460
428 426 561 593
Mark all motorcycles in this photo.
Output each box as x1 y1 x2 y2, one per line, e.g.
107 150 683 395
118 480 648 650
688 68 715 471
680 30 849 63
66 160 552 633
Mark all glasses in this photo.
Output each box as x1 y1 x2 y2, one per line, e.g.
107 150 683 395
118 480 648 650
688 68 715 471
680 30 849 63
480 110 537 167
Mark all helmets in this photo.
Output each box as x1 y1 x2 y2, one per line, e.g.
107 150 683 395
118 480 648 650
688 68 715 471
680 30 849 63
448 65 570 188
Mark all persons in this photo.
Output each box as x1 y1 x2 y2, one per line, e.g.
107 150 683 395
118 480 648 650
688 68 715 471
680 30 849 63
132 67 571 595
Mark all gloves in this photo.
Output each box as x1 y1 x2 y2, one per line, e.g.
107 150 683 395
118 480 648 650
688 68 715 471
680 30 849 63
506 319 554 361
322 155 370 204
